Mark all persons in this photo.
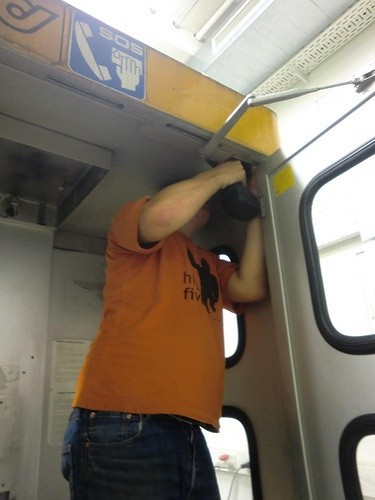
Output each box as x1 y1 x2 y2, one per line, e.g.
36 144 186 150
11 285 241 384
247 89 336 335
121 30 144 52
59 160 272 500
